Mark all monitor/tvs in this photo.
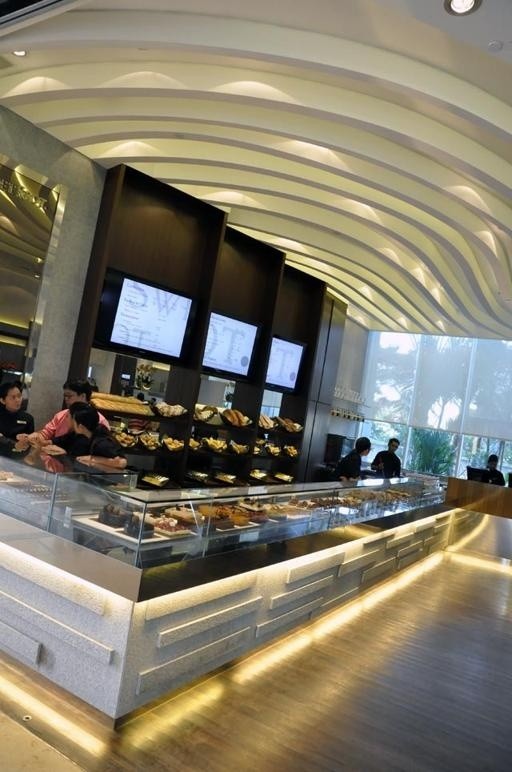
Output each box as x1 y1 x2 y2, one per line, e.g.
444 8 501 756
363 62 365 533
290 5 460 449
468 467 490 484
91 267 208 370
261 333 309 396
198 309 262 382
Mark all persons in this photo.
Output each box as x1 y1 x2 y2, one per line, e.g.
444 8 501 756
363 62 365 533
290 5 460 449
326 437 371 485
371 438 401 478
486 454 505 486
0 380 34 456
27 377 127 468
128 393 151 431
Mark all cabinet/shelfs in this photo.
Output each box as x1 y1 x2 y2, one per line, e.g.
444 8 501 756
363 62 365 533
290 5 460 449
87 390 304 489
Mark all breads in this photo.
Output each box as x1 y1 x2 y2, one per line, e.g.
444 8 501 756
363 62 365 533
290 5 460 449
90 392 302 486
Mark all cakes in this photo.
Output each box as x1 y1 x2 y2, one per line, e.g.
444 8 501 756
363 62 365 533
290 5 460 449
0 470 69 500
100 489 412 539
